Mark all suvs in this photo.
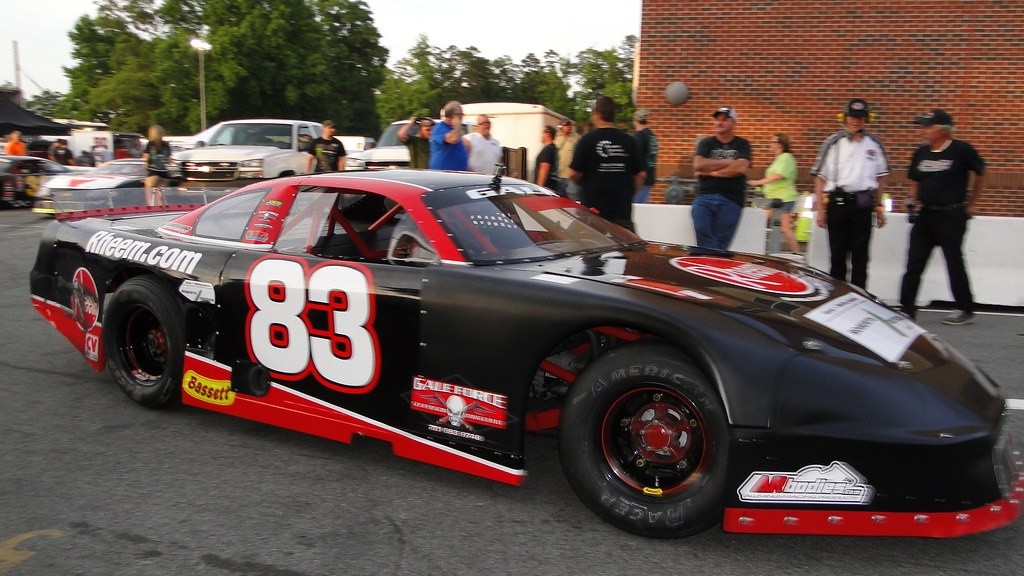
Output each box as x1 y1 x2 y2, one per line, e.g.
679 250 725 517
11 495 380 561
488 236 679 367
174 119 325 186
345 115 469 176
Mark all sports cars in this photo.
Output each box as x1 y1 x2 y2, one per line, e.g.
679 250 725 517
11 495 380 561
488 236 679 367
23 171 1024 537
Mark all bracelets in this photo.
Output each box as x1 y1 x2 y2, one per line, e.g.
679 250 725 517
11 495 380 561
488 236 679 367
712 171 717 176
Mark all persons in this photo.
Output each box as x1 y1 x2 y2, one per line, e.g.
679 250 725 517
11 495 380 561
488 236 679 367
48 138 75 166
5 131 26 155
143 125 171 206
809 97 890 291
534 126 559 190
304 120 347 174
747 132 800 253
429 101 468 171
569 96 642 230
398 115 436 169
632 108 658 203
691 107 752 250
553 119 580 196
77 142 109 167
899 110 985 325
462 114 503 175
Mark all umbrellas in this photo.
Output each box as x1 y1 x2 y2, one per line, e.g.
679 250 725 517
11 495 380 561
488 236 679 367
0 95 71 137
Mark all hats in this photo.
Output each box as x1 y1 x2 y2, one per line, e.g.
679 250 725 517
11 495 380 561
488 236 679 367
845 99 868 116
914 109 952 126
713 107 737 121
323 120 336 128
558 118 573 126
631 110 648 120
420 117 435 126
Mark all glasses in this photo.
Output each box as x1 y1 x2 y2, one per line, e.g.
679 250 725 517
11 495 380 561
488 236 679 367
479 122 490 125
455 113 464 118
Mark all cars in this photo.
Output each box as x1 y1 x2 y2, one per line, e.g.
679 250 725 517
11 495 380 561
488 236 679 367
32 157 179 217
0 155 77 208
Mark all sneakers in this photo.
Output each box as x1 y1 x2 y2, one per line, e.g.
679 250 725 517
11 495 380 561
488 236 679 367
942 309 976 325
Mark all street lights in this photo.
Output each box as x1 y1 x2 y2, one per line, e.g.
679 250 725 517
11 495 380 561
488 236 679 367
190 38 217 132
